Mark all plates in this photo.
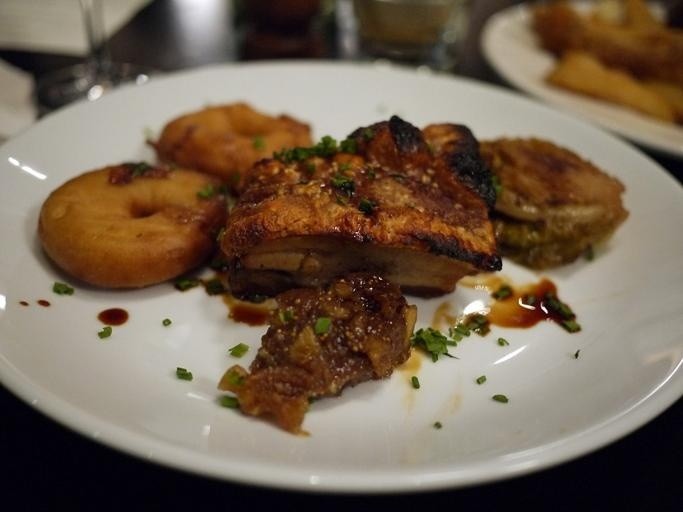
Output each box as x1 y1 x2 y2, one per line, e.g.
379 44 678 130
1 1 152 54
3 59 683 495
477 5 683 157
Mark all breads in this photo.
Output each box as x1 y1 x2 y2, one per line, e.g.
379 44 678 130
38 162 228 291
154 104 313 198
